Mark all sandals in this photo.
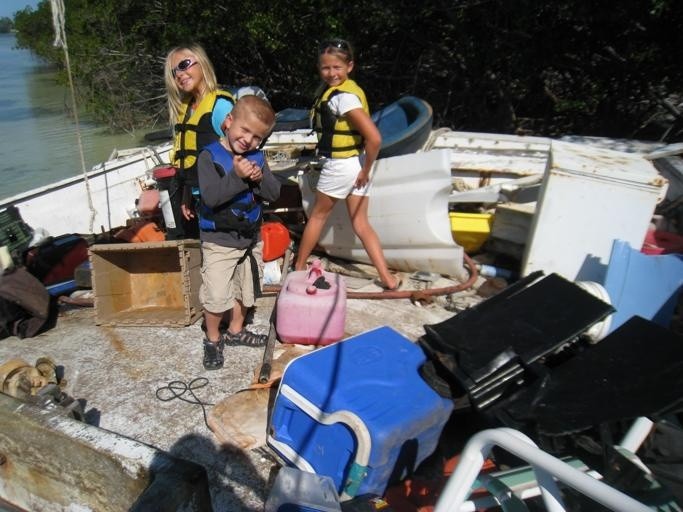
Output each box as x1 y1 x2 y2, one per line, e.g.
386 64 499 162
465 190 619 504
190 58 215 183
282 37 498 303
225 329 267 347
204 334 224 370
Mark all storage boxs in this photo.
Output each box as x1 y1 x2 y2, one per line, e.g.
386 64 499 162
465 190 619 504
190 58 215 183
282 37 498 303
87 238 206 328
261 325 455 502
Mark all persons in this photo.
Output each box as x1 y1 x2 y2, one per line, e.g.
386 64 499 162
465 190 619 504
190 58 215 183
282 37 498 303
196 95 281 370
164 41 234 241
293 37 400 289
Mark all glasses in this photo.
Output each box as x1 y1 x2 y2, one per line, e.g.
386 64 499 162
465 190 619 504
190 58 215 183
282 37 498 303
172 58 197 78
318 39 346 49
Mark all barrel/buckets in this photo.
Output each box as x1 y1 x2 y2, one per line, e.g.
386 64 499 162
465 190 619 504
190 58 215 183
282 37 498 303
260 220 291 262
526 279 612 344
